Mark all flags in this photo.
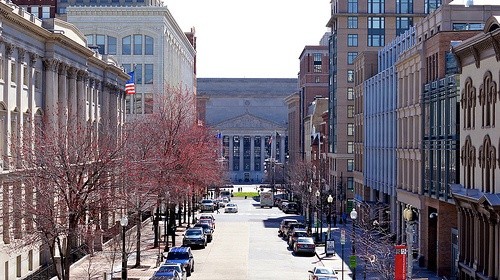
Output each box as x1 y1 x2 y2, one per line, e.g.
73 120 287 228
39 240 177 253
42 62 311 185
126 71 135 94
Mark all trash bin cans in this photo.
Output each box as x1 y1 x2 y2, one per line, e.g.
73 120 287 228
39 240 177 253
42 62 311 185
192 212 198 223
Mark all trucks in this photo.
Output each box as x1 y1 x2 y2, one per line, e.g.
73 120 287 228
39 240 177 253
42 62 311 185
260 192 274 208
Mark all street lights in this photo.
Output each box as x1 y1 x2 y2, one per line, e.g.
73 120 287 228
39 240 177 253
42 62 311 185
118 215 129 279
315 178 325 242
164 190 171 252
327 170 343 278
349 197 370 280
286 149 307 228
307 179 315 236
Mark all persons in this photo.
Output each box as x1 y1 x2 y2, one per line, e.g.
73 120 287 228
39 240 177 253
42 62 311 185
216 203 220 214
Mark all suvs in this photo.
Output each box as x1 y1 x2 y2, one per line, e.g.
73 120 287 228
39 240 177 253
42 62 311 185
201 199 215 212
165 246 194 277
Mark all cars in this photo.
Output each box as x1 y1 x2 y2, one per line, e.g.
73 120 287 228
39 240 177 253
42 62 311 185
280 218 315 256
199 214 216 231
274 197 298 214
151 269 180 280
181 227 206 248
195 223 212 243
211 197 229 209
225 204 237 213
309 266 339 280
159 263 187 280
